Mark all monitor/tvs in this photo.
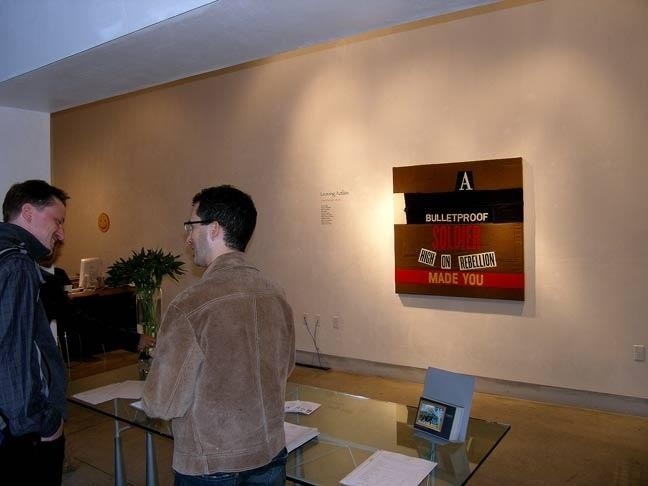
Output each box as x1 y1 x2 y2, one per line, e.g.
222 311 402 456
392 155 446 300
79 259 104 289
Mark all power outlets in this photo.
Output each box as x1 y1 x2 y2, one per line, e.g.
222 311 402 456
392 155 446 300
632 345 646 362
331 314 339 329
302 312 309 326
313 314 319 328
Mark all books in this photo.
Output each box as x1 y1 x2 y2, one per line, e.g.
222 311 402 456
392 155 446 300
285 422 319 454
413 396 465 441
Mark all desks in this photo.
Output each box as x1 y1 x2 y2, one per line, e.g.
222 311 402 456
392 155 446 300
62 358 510 486
55 285 138 364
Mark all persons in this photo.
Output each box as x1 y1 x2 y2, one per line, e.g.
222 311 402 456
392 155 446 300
0 179 71 485
150 184 296 486
39 233 156 353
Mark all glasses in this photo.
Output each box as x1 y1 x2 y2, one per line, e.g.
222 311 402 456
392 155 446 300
184 220 207 233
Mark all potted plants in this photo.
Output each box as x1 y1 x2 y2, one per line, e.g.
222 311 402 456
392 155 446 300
103 248 186 379
